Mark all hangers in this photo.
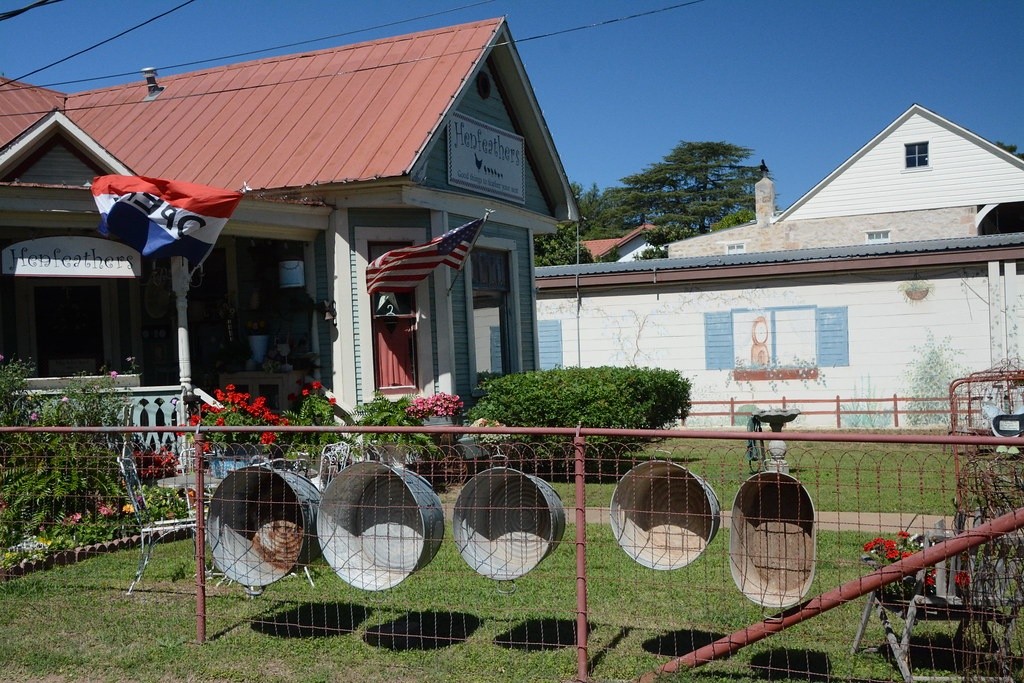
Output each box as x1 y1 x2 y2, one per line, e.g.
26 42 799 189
383 305 398 324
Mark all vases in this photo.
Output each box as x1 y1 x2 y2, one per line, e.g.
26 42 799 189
423 416 454 427
207 454 270 479
458 437 497 460
248 335 270 371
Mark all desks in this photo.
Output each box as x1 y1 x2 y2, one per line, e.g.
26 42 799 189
156 473 315 590
218 370 308 411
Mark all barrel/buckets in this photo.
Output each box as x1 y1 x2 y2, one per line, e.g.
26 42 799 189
205 465 323 596
730 459 815 622
452 454 565 596
608 450 720 571
316 459 445 593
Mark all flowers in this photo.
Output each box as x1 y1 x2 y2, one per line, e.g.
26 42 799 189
861 530 969 596
406 391 464 419
30 412 38 420
99 506 116 516
135 445 180 483
281 380 336 445
175 384 290 454
108 370 118 379
463 417 511 443
62 512 81 529
247 318 271 335
122 503 135 514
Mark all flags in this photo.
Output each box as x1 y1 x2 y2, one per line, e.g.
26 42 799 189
366 208 496 296
91 173 252 278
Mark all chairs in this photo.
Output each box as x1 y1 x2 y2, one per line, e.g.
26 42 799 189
319 441 350 493
115 456 206 596
180 447 224 544
873 506 1024 683
